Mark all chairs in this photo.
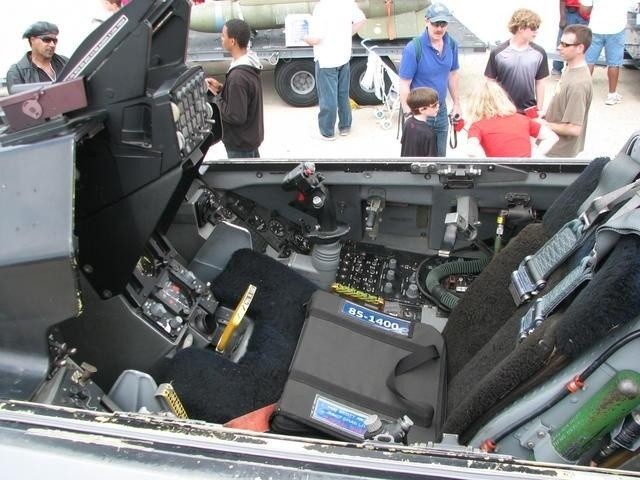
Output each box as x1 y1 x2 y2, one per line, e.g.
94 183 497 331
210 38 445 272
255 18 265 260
169 124 639 465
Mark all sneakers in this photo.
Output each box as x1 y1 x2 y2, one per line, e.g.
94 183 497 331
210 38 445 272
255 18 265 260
604 93 624 105
340 129 350 136
320 134 336 141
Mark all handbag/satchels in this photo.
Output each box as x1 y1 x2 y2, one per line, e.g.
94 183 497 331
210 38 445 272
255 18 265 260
268 288 449 445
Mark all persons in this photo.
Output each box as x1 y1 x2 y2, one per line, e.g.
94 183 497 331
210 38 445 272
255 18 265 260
103 0 121 13
398 2 461 157
400 86 441 158
542 24 592 159
462 76 560 160
5 20 71 95
556 0 593 51
584 1 630 105
485 6 549 115
301 0 367 140
205 19 263 159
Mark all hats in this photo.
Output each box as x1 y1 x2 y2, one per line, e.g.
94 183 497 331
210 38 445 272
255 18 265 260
424 3 452 24
21 21 59 40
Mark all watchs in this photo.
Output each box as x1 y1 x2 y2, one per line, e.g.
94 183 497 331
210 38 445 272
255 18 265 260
403 112 414 119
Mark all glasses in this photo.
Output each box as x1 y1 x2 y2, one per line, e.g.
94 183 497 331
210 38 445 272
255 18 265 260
527 26 540 31
414 100 440 111
35 36 58 45
560 40 579 48
